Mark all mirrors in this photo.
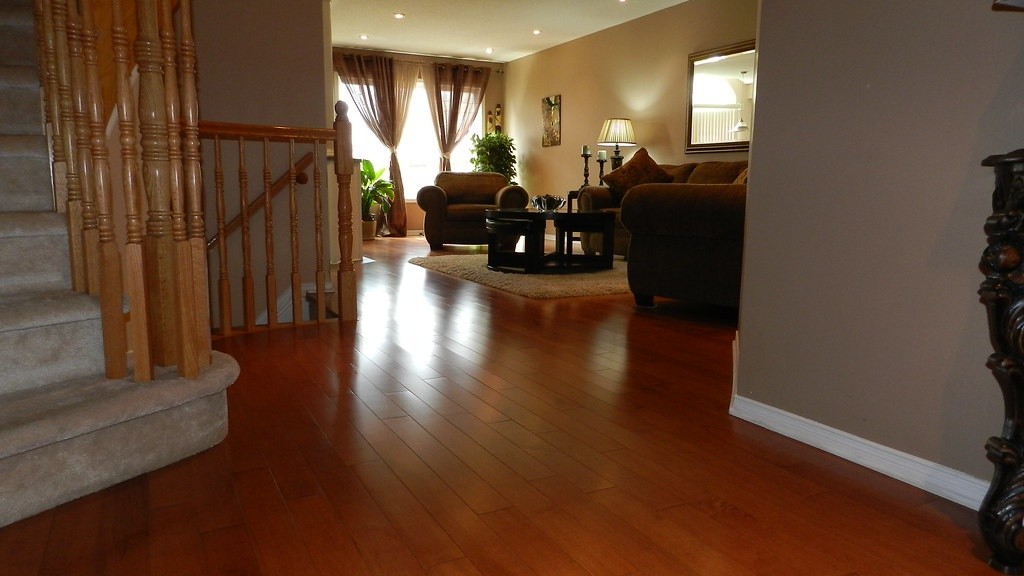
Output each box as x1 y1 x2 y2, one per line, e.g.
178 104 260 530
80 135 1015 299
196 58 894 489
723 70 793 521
685 39 757 154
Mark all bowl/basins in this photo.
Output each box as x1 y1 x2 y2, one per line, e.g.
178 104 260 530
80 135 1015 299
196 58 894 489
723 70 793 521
532 199 566 212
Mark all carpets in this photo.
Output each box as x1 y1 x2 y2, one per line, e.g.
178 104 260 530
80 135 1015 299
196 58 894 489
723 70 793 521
407 254 631 297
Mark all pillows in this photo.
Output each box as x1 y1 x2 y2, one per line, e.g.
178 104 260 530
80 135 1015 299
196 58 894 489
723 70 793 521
601 146 674 203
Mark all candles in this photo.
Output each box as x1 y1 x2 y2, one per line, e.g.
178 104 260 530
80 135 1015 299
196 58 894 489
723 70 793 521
582 145 592 154
597 150 606 160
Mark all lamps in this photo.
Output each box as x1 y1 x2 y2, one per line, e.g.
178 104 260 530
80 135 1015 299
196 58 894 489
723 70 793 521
731 71 749 132
597 117 637 171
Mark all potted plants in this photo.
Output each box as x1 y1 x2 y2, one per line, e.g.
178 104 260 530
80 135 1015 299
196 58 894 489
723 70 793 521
359 160 397 240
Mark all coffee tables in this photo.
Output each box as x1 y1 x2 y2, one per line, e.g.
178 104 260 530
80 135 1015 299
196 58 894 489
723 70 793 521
486 208 616 274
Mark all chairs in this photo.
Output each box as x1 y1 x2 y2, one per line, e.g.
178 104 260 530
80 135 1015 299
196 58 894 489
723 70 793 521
416 171 530 251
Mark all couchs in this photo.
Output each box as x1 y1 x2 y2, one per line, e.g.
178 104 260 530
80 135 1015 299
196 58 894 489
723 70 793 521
577 161 748 307
